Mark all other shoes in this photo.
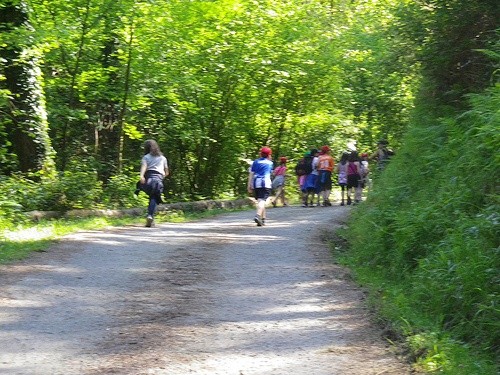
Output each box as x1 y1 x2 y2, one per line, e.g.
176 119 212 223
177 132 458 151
254 215 266 226
145 216 153 227
283 203 287 206
272 201 276 207
353 201 361 205
301 201 332 207
340 202 344 206
347 201 351 205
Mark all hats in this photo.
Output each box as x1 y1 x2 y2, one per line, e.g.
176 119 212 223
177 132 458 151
311 149 320 153
322 145 330 151
377 139 389 144
261 147 272 155
280 157 286 162
361 153 368 157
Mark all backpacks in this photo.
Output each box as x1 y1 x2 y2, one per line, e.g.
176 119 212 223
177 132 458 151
295 157 313 175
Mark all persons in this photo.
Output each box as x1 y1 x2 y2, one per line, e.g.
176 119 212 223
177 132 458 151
271 156 288 207
295 139 393 207
247 146 274 227
135 139 169 227
